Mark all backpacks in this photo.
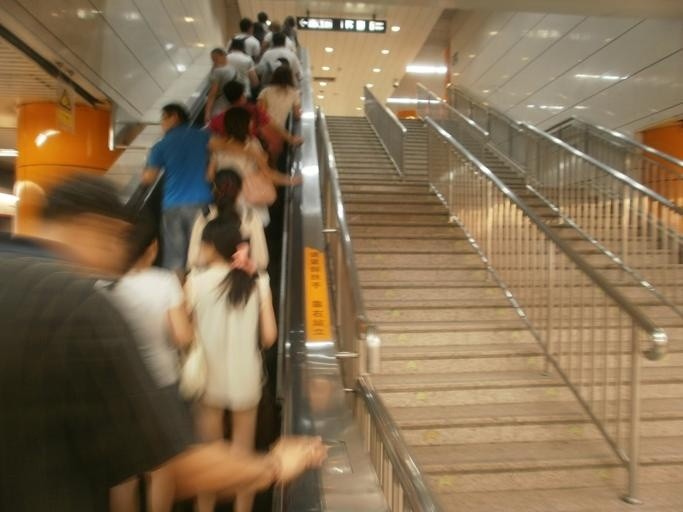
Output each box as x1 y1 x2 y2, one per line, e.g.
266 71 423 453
228 34 253 52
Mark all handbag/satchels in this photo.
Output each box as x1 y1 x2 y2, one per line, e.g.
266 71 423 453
176 327 208 404
241 170 278 207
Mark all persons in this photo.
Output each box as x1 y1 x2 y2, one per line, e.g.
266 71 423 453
1 10 329 512
